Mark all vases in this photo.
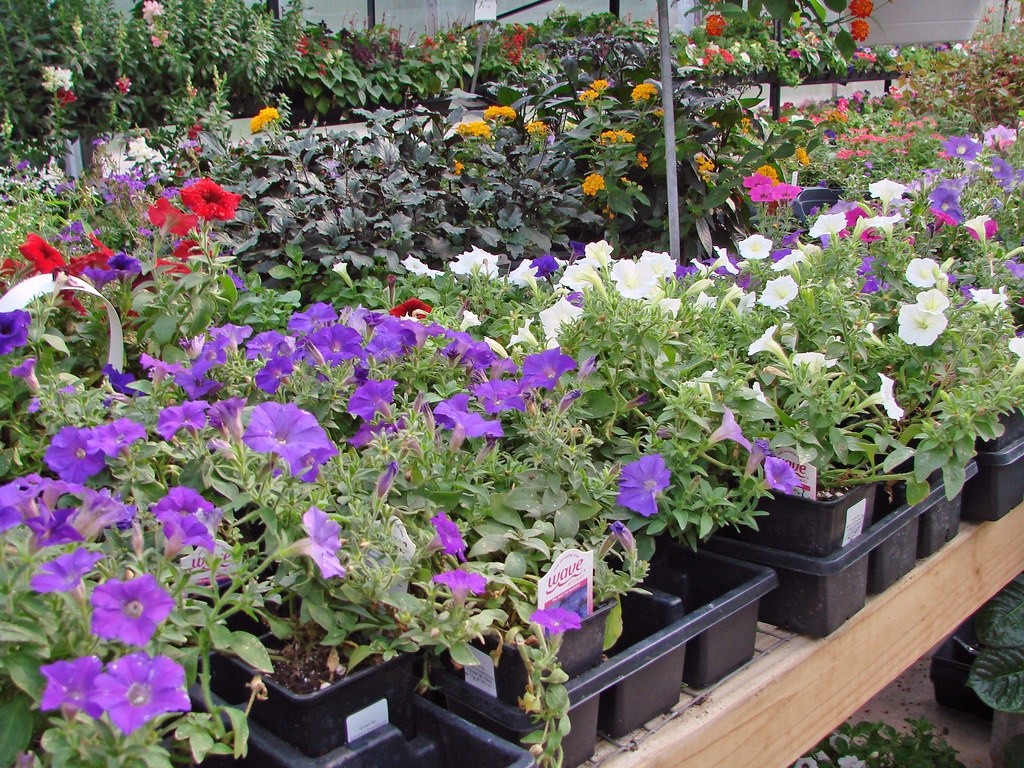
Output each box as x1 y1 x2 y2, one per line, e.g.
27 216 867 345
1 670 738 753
745 484 877 559
153 645 537 768
905 409 1024 524
186 566 427 756
421 546 779 768
654 460 977 639
929 656 985 715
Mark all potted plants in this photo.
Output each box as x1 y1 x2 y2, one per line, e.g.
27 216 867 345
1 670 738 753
965 572 1024 768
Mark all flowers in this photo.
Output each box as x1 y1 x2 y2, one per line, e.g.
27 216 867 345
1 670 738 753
0 0 1024 768
